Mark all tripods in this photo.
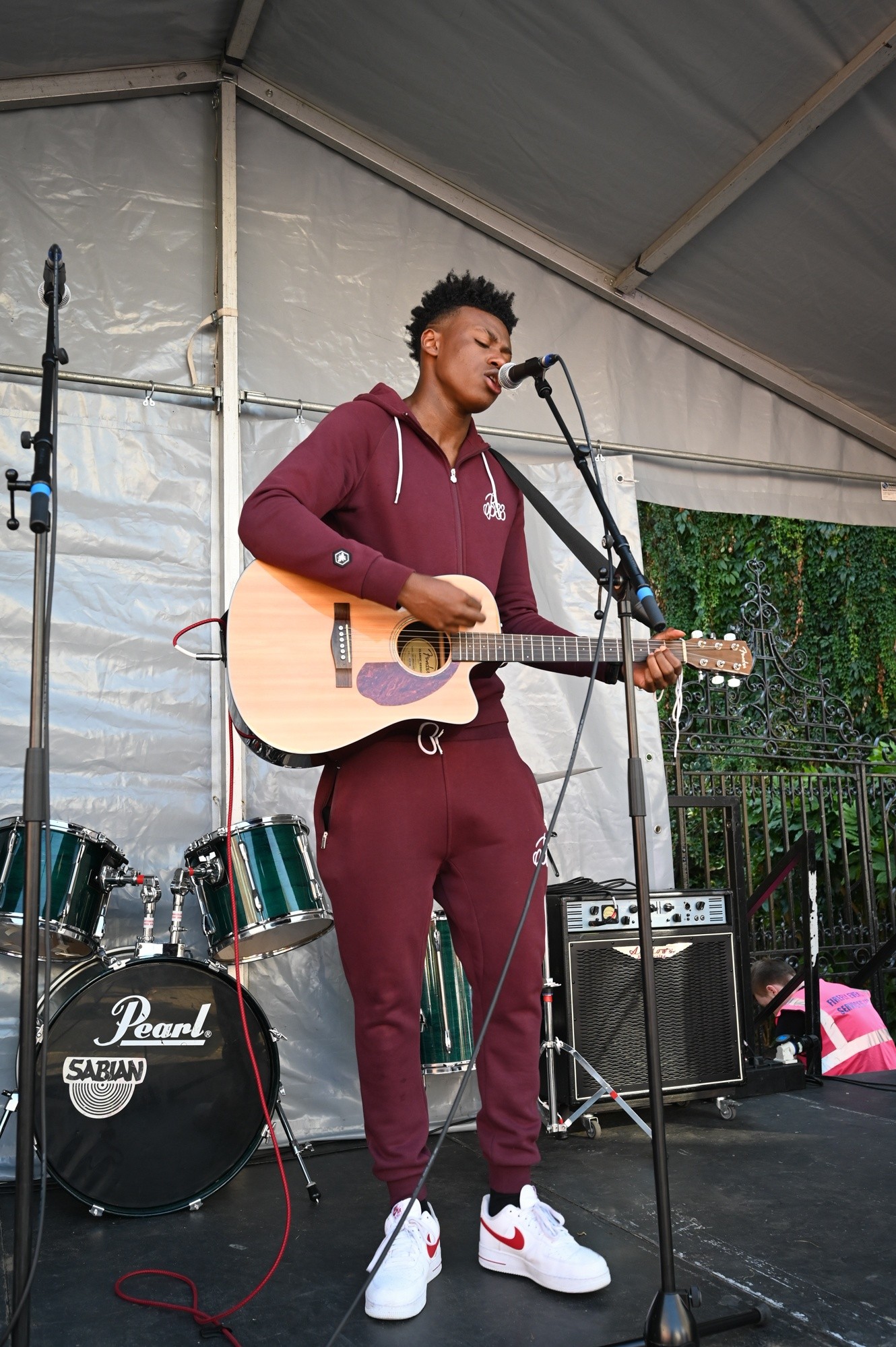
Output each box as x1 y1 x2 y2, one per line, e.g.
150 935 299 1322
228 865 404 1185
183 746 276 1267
539 898 652 1137
524 356 770 1347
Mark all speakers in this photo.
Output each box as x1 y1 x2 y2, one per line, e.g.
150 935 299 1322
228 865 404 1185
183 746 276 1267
546 889 750 1115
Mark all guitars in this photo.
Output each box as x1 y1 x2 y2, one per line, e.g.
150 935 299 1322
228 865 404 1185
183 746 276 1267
222 556 755 770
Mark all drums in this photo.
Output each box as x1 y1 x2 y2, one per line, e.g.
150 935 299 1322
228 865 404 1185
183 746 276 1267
417 909 478 1074
15 942 286 1221
0 814 126 962
184 812 336 965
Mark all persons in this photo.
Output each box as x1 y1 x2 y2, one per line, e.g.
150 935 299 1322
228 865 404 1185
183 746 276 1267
749 958 895 1076
239 267 687 1321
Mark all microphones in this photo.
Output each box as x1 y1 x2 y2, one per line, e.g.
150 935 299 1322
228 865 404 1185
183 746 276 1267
37 244 71 310
498 354 558 391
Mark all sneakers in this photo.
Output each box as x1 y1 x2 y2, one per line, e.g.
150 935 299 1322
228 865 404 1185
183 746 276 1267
477 1183 612 1293
366 1196 443 1320
